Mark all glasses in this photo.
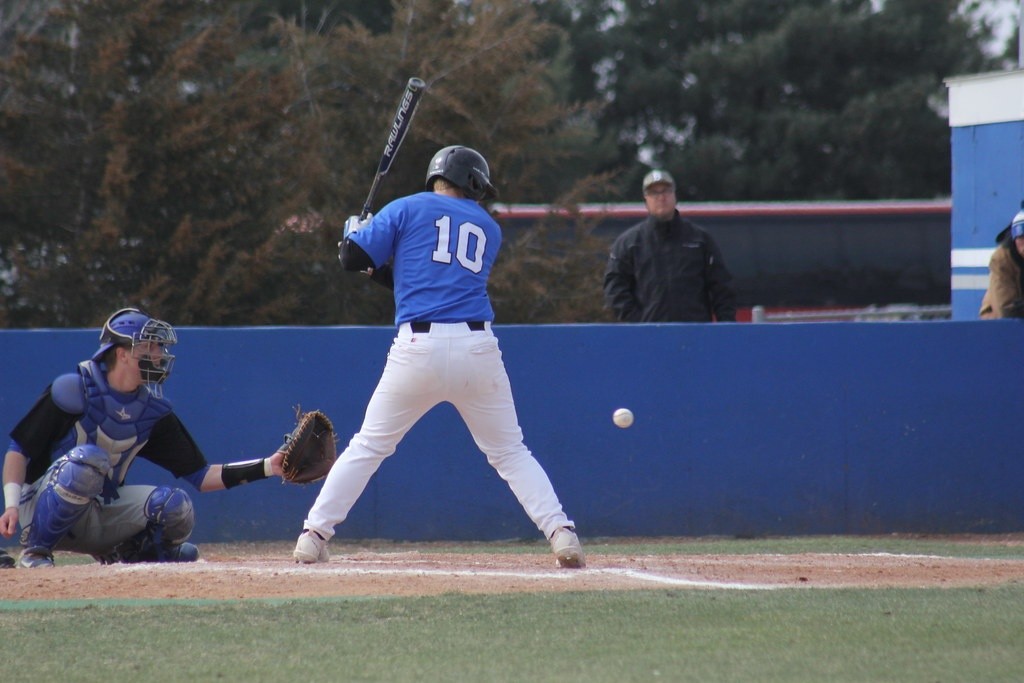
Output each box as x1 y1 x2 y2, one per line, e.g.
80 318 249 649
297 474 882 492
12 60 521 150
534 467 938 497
642 187 673 198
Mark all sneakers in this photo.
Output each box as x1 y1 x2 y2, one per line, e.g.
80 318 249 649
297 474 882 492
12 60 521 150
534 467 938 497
293 529 331 564
550 528 587 569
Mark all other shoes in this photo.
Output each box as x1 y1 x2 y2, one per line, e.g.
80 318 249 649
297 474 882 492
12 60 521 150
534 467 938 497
19 553 53 569
170 542 201 563
0 547 15 566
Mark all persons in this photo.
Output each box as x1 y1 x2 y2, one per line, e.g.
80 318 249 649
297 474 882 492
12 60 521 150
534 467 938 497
979 209 1024 319
294 145 585 569
0 306 337 568
602 168 736 323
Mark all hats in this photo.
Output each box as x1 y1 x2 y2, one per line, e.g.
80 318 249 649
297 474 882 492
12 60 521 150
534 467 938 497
1011 212 1024 241
644 170 677 192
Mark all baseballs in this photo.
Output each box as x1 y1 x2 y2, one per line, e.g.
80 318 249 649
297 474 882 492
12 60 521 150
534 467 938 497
613 408 634 428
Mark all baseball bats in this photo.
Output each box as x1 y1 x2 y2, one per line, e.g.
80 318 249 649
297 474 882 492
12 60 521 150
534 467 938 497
359 76 426 221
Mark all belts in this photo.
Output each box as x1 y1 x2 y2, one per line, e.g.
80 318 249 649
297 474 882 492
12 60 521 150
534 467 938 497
410 320 486 333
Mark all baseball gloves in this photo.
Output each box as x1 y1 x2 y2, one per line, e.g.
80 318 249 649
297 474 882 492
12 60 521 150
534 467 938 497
276 402 342 488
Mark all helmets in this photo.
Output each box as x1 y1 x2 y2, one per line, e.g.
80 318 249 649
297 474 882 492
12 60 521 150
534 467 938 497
424 145 499 201
92 309 156 362
